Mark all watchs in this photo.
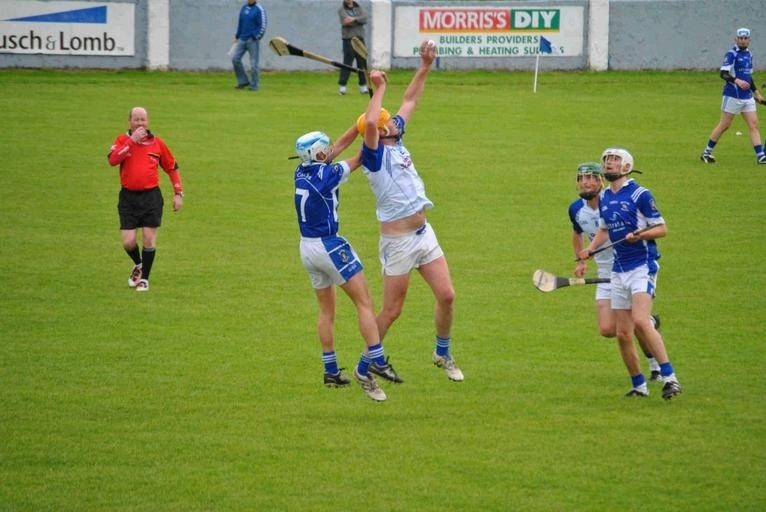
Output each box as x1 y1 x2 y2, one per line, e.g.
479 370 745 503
175 192 184 197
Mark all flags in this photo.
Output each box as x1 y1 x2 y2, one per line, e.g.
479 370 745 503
538 36 560 55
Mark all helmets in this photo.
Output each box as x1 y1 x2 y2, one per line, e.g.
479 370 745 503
736 28 750 39
295 131 333 162
577 148 633 200
357 107 391 138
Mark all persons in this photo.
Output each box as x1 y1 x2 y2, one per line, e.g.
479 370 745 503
567 161 663 385
337 0 369 95
107 107 184 292
352 40 465 402
700 27 766 165
294 123 405 388
229 0 267 91
577 148 682 401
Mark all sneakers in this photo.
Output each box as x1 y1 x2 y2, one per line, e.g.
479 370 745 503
757 152 766 163
340 84 369 95
701 150 715 163
324 361 403 402
624 366 682 400
432 349 464 381
128 263 149 291
